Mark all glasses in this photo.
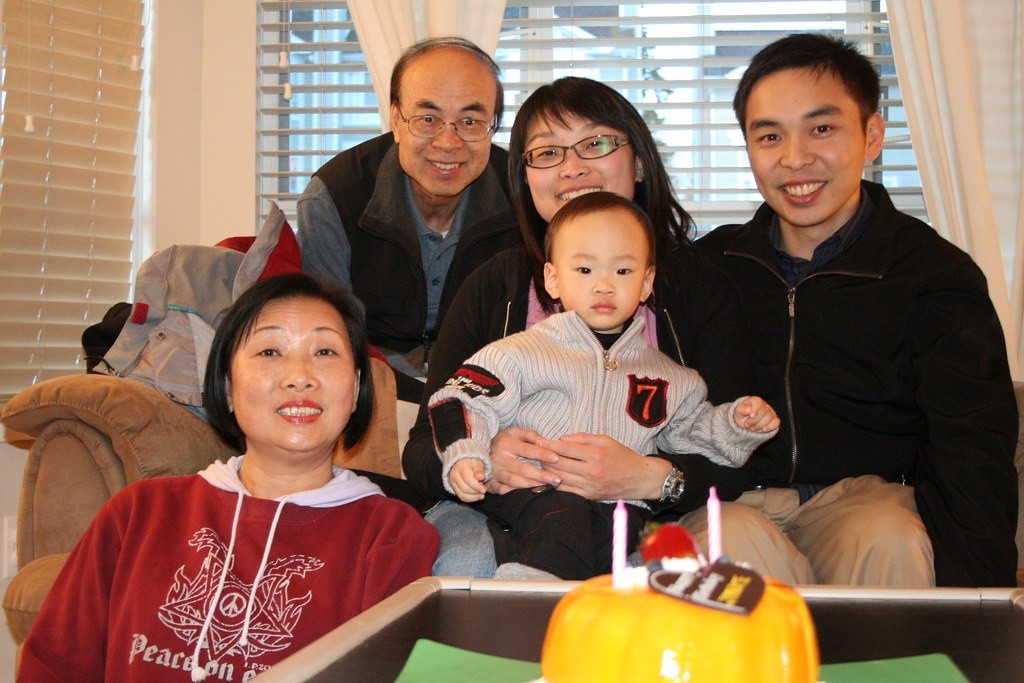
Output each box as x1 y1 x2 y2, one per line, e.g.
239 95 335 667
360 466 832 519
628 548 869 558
399 107 497 142
520 135 633 170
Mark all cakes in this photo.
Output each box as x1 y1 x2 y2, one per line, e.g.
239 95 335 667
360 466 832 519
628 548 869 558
542 521 820 683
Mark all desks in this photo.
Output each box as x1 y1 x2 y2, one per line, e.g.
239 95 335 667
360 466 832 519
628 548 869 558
245 575 1024 683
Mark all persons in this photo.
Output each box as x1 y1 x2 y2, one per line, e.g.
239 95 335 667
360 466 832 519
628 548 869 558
293 34 525 579
402 75 697 580
424 192 782 580
691 32 1020 590
15 273 441 683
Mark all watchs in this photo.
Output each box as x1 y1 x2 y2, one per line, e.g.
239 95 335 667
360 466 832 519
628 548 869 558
659 465 685 504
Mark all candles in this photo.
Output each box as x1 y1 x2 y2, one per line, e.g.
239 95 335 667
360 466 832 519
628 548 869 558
707 486 723 564
611 500 627 589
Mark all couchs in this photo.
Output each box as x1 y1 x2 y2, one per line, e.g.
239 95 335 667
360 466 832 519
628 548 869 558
0 372 1024 683
